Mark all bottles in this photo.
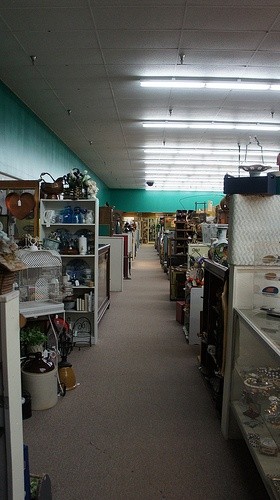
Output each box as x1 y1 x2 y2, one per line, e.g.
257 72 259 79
78 235 87 255
21 351 58 412
44 206 94 224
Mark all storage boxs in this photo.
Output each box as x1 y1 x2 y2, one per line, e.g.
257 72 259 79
224 172 277 196
176 301 185 323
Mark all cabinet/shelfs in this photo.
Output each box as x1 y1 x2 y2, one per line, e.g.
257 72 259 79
0 178 138 500
139 217 159 244
158 193 280 500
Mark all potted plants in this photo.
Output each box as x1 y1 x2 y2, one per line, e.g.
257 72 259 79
19 326 48 355
